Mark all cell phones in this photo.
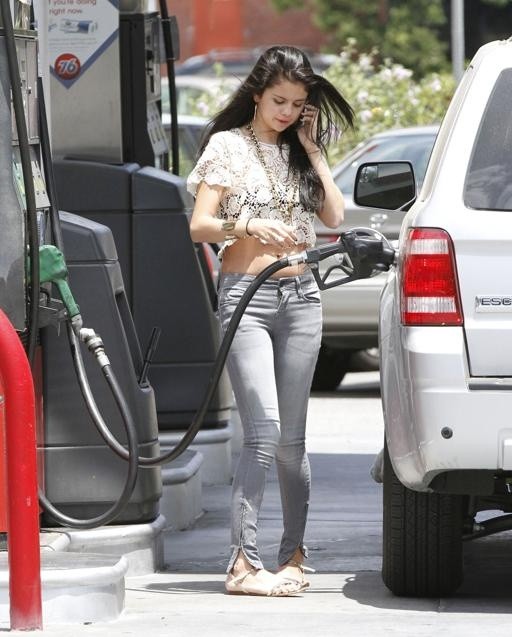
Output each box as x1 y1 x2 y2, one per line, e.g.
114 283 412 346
295 103 307 127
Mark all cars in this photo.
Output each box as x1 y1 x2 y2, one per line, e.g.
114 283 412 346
310 126 439 392
160 49 343 176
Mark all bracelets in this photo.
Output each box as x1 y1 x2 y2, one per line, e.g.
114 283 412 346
307 149 321 155
222 217 240 241
246 217 253 237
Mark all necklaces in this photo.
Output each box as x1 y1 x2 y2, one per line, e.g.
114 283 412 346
248 122 300 227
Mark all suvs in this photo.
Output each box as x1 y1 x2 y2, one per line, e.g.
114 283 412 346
352 37 512 599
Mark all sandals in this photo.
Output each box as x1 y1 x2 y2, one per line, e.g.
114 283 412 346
224 568 298 596
274 559 316 596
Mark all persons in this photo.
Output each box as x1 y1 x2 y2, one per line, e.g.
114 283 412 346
186 45 356 597
